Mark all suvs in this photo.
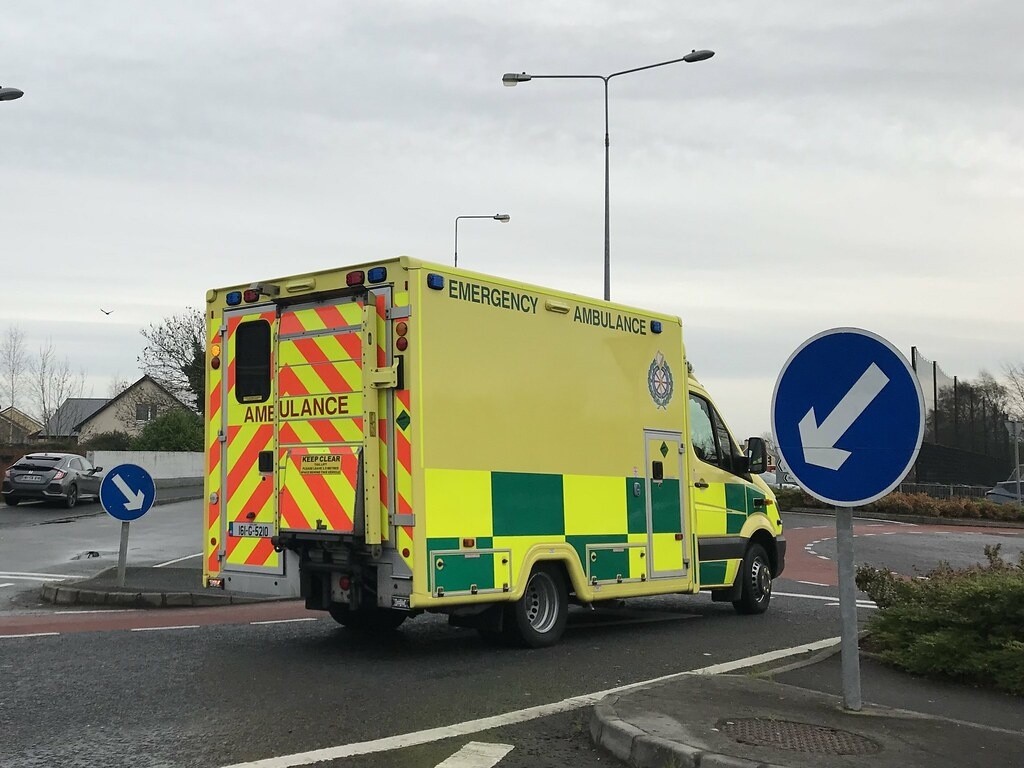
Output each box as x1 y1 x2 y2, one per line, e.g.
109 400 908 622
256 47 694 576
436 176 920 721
985 481 1024 504
1 453 105 509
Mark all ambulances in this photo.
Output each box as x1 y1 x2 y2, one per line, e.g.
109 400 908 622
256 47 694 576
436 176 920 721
206 256 787 648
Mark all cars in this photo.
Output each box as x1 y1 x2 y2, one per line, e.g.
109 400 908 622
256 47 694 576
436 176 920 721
757 470 800 491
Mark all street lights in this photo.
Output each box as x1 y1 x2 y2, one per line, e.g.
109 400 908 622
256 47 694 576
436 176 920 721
502 49 715 302
454 214 510 268
